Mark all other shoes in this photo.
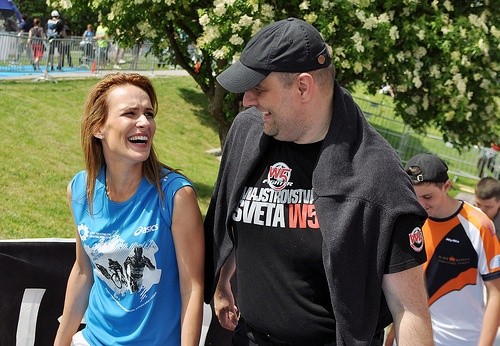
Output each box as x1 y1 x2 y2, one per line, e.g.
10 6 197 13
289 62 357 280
119 59 126 63
12 61 19 64
69 65 73 67
57 66 61 69
114 65 121 69
33 63 37 70
51 66 54 70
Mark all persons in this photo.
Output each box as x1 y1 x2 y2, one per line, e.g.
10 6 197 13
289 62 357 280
45 11 73 71
28 18 45 70
14 15 33 63
53 72 204 346
384 153 500 346
473 177 499 243
81 21 126 69
203 17 433 346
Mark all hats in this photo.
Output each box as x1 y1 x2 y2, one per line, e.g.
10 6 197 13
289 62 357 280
51 10 60 17
216 17 331 93
405 153 449 183
